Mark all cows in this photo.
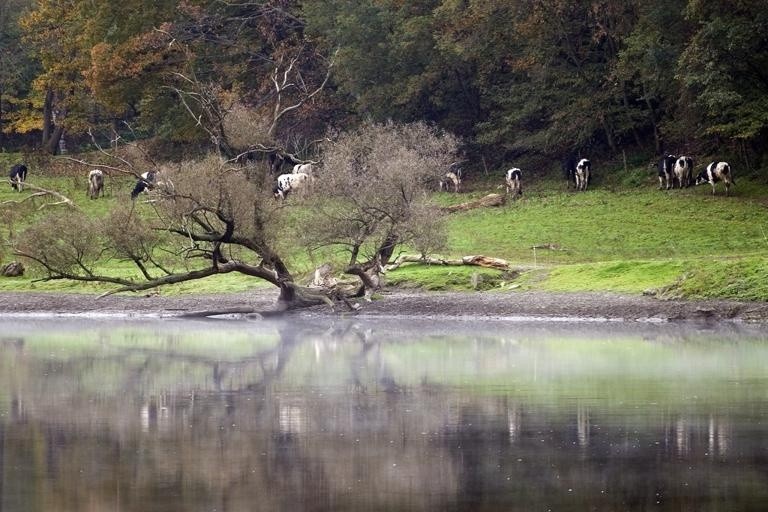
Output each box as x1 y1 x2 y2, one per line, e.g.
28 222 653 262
291 162 320 173
9 163 28 193
652 153 694 191
564 156 591 191
695 161 737 197
86 169 105 199
439 162 463 193
129 166 167 200
505 167 523 198
273 172 312 203
